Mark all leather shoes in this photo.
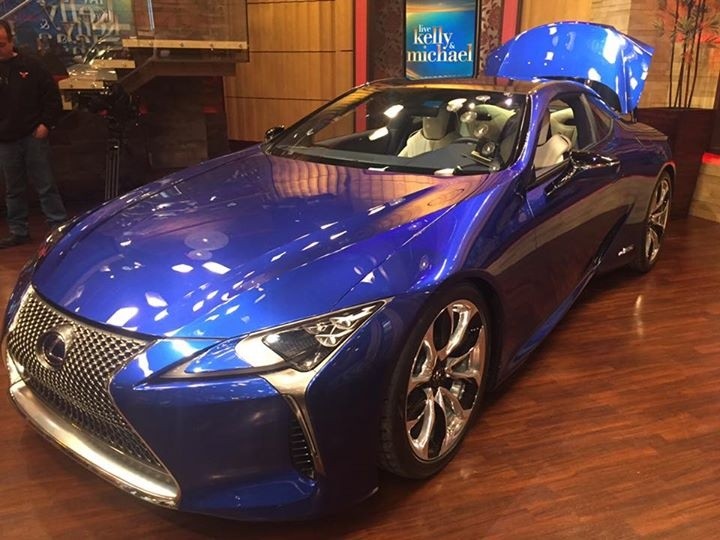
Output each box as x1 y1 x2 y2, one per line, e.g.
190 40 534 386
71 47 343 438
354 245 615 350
0 233 29 248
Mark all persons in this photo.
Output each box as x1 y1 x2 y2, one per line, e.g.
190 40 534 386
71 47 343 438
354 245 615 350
44 51 151 190
0 19 69 248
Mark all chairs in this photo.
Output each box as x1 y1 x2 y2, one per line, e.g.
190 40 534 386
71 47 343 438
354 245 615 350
396 98 578 178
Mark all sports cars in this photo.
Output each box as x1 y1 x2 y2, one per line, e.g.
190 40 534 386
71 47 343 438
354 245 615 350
1 20 683 526
